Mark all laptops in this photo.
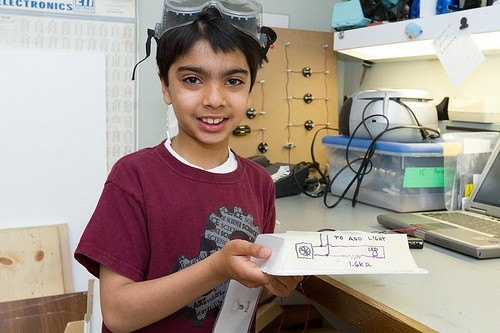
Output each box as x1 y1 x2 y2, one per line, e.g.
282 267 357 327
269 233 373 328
376 136 500 260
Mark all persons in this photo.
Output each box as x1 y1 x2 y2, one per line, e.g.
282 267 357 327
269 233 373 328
72 0 305 333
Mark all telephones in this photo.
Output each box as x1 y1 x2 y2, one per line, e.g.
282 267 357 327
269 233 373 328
245 155 309 198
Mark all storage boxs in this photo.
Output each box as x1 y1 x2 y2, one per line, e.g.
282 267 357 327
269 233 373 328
322 134 462 213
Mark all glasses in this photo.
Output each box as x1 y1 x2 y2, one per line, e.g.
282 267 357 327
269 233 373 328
153 0 265 48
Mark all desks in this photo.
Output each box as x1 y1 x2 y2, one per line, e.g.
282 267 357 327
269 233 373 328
274 190 499 332
